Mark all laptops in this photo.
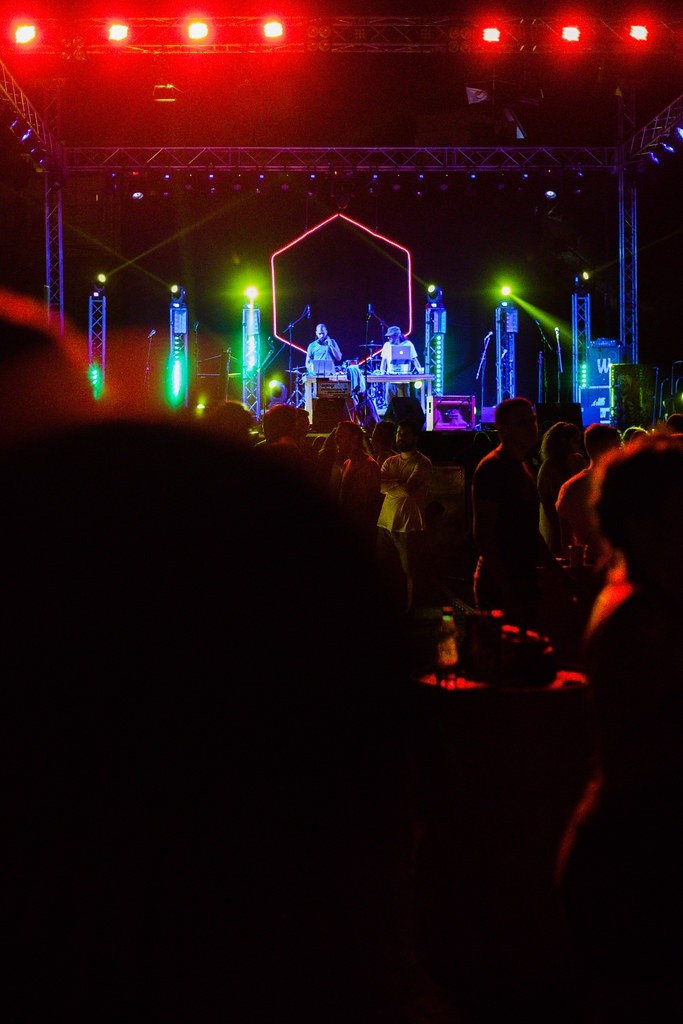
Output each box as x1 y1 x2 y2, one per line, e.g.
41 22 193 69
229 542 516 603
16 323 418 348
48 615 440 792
390 345 411 364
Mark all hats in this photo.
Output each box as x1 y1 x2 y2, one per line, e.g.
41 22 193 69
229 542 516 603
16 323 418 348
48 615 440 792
385 327 401 336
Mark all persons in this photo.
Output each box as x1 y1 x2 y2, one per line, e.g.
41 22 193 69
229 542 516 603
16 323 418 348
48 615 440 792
0 291 683 1024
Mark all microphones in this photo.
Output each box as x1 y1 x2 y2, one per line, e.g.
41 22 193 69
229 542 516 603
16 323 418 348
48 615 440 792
193 323 199 330
148 330 156 339
368 304 372 314
555 327 559 335
308 305 311 319
484 331 493 340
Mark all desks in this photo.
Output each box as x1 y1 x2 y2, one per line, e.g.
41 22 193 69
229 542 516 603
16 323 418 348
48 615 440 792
302 374 435 424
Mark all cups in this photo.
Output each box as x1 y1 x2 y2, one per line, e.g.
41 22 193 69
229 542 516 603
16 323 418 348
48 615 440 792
569 545 588 568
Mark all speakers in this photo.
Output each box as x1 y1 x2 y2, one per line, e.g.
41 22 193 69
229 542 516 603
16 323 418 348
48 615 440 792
434 395 475 430
586 345 631 386
534 402 583 431
481 407 497 431
313 397 353 433
383 396 426 434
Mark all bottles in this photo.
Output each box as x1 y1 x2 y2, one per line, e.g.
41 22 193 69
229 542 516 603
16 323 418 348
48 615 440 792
436 606 458 689
